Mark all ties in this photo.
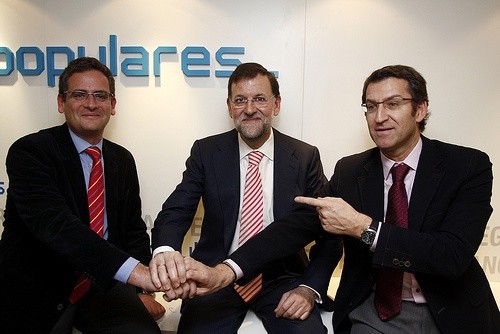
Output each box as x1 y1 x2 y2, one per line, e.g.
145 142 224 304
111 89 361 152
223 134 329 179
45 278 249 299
232 151 265 303
68 146 105 305
374 164 410 322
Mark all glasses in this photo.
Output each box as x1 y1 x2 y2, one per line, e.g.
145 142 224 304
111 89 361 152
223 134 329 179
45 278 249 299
228 93 276 108
60 89 115 103
360 95 422 113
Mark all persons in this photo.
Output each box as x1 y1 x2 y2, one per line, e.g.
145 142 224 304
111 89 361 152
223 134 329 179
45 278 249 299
0 57 196 334
149 62 343 334
166 66 500 334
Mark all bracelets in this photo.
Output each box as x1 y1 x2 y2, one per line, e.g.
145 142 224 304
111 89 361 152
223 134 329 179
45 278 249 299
220 261 236 283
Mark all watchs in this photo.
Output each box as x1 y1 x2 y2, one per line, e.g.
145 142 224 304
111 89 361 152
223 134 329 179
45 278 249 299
137 288 155 296
360 218 379 247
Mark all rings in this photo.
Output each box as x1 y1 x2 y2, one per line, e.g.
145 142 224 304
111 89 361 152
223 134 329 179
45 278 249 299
157 264 166 267
